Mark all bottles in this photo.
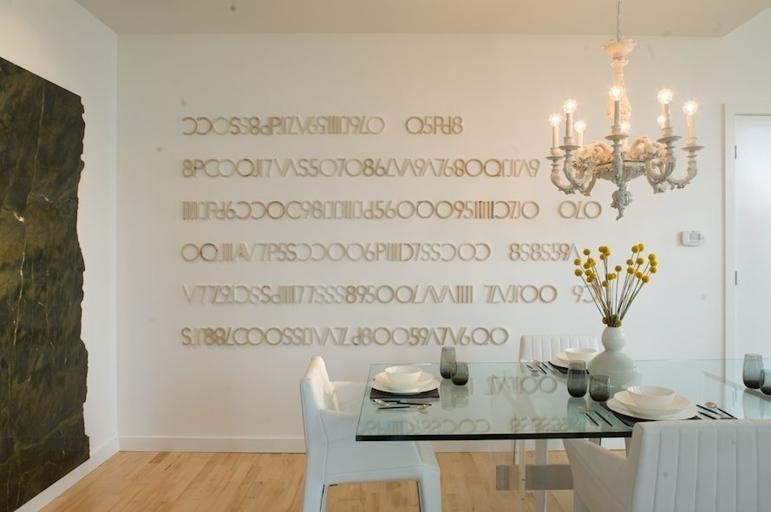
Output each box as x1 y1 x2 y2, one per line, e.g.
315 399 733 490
566 360 587 398
743 352 765 389
440 347 458 378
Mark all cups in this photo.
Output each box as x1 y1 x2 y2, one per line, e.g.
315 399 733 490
760 370 771 396
590 374 610 401
449 363 470 387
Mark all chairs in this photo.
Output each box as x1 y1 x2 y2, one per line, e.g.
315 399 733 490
300 356 441 512
563 420 771 512
514 333 599 512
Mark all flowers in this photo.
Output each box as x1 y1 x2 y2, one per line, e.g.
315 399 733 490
574 243 658 327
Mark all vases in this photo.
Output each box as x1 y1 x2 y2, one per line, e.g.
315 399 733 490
588 327 638 388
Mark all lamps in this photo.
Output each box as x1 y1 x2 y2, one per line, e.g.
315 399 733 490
546 0 705 220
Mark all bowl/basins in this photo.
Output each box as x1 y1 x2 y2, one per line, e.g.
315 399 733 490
627 386 675 407
565 347 595 359
386 364 421 382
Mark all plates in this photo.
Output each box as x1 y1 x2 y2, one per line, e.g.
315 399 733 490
548 354 586 370
606 389 700 419
370 373 441 394
